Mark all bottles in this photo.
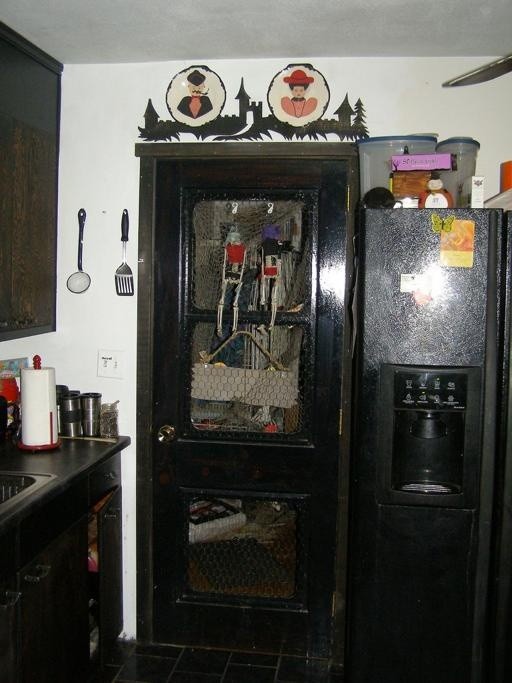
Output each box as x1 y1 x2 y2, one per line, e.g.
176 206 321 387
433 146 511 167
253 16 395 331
101 404 118 437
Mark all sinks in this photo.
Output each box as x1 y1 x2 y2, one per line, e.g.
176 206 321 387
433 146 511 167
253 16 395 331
0 470 58 515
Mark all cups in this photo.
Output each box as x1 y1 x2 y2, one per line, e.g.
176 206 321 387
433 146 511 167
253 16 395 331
56 384 102 438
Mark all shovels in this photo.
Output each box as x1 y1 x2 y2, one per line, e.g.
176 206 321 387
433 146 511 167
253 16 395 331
115 209 135 297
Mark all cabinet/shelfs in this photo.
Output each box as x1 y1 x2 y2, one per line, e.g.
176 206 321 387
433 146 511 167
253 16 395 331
0 450 123 682
0 20 64 343
135 140 358 661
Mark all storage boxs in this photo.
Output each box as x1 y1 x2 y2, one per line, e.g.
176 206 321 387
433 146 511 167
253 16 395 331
356 136 480 208
